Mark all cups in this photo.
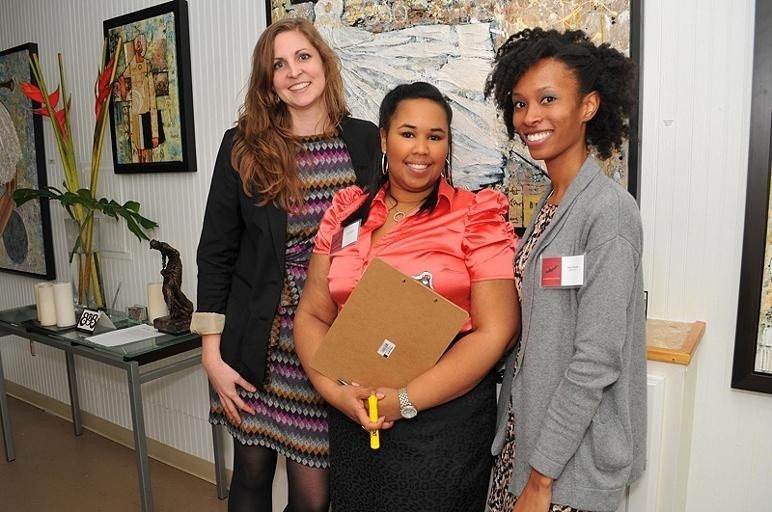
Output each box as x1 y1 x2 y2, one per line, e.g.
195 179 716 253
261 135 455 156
53 283 77 327
33 281 56 326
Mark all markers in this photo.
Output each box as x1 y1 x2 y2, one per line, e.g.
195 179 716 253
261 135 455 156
368 395 380 449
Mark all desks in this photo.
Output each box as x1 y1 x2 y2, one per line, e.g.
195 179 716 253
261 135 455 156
0 306 227 512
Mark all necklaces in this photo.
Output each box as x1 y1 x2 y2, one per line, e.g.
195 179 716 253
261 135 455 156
381 196 427 222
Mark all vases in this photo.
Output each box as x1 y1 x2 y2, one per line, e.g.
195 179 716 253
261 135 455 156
63 218 106 308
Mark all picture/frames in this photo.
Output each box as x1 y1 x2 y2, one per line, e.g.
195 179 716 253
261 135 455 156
100 0 199 174
0 42 55 282
264 2 642 237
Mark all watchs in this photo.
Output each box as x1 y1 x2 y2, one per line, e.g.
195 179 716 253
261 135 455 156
396 388 420 421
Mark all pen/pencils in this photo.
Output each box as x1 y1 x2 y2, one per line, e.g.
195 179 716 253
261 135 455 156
337 378 348 385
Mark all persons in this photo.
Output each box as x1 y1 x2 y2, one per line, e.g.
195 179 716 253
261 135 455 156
294 80 521 511
190 19 383 512
484 28 646 511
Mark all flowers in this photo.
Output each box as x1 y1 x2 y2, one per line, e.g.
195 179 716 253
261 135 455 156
5 37 156 306
